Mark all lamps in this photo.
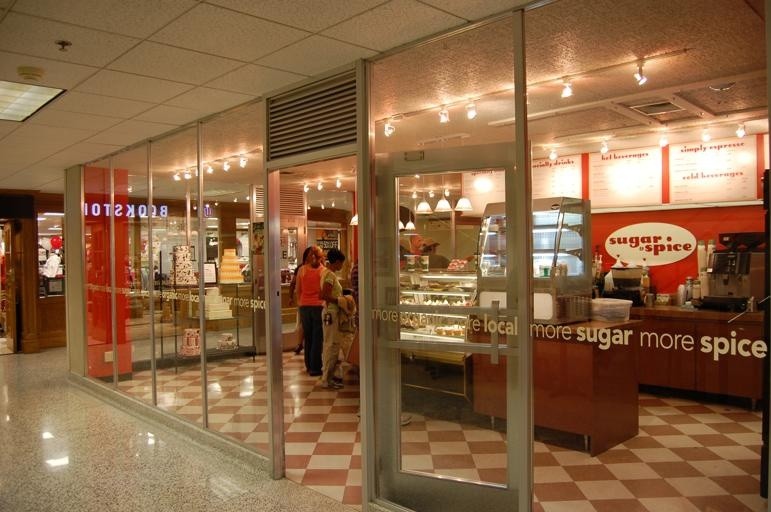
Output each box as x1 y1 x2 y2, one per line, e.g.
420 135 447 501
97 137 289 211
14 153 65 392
350 168 359 225
542 116 767 160
373 52 690 136
299 172 355 193
172 145 263 181
398 171 473 232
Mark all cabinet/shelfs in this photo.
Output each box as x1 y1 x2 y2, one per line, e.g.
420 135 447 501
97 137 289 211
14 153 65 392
401 265 479 426
477 197 591 326
165 283 256 373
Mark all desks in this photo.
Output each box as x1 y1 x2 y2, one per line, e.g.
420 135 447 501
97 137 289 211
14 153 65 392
471 318 644 457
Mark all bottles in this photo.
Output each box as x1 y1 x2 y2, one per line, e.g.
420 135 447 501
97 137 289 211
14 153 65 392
678 240 715 307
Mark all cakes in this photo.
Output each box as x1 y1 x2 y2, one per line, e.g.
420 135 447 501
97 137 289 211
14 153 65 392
219 249 246 284
196 287 233 320
168 245 197 285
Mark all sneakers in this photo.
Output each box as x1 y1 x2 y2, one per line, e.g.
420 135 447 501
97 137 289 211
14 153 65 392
293 344 344 391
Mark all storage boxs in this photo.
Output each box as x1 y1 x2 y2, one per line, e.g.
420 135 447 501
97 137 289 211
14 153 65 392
594 298 633 322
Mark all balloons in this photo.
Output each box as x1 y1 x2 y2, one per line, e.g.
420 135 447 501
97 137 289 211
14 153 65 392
51 236 63 249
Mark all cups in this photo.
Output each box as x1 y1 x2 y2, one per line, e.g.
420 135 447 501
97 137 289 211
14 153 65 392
644 294 654 307
407 256 415 271
421 256 430 270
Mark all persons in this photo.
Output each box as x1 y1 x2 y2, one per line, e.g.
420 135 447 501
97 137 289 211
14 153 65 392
401 414 413 425
421 236 452 269
317 247 357 390
287 246 313 353
295 245 328 376
410 233 424 255
400 242 412 271
